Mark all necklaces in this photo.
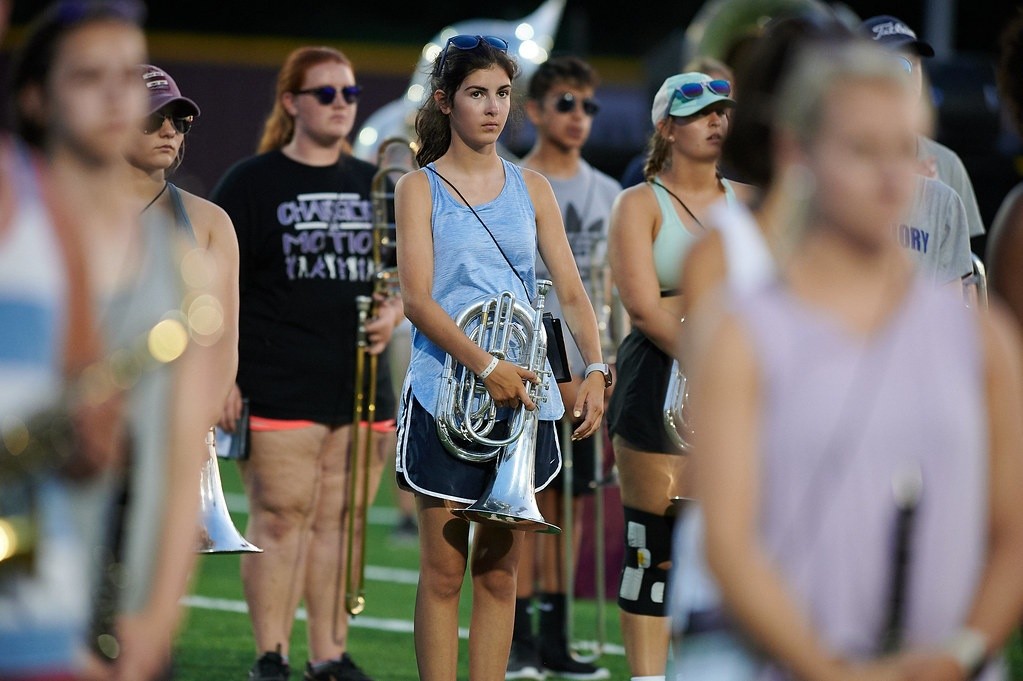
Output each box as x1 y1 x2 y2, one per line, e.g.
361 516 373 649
646 175 705 232
571 168 595 250
139 180 168 216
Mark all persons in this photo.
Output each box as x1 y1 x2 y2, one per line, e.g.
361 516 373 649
660 42 1023 680
857 17 985 316
0 0 239 681
203 33 763 681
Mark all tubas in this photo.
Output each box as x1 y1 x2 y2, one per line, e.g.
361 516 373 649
199 421 268 559
659 354 696 507
435 276 564 536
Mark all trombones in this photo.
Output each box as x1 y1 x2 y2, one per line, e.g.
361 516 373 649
342 134 421 620
562 258 624 664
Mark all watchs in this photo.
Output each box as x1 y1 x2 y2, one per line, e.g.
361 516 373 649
585 363 612 389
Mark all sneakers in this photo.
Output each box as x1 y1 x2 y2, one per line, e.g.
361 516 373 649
247 651 293 681
303 652 372 681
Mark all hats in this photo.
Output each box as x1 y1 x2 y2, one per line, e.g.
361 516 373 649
650 72 739 132
130 64 201 123
864 14 937 60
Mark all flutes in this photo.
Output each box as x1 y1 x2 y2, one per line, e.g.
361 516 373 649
880 504 920 657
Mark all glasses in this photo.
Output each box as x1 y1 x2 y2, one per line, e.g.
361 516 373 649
557 92 603 118
663 79 731 123
289 85 363 106
435 34 508 80
140 105 194 133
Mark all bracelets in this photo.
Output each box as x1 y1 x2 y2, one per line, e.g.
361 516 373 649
945 626 987 675
479 357 499 379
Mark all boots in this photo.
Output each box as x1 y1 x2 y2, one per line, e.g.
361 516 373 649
539 589 610 679
505 596 549 679
